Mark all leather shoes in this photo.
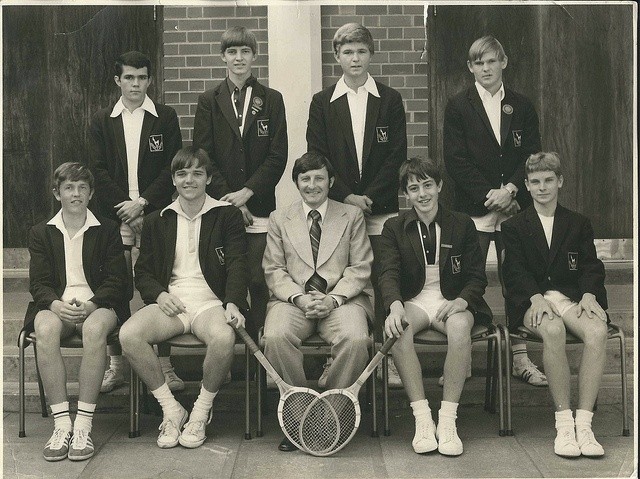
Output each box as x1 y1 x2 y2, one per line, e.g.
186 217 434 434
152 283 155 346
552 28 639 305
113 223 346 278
278 423 301 450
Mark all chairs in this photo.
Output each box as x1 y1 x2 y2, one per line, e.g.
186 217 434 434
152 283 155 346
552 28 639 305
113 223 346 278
135 249 254 440
19 246 135 438
382 245 503 436
258 244 378 437
501 247 629 436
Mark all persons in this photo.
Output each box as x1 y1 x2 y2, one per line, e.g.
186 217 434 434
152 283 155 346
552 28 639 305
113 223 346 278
436 34 547 385
262 151 375 453
374 157 487 455
26 163 128 461
86 50 184 392
193 26 288 392
118 147 249 448
307 24 408 389
499 152 611 457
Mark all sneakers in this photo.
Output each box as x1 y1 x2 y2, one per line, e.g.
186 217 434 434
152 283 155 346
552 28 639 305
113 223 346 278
162 367 184 390
412 419 438 453
512 357 549 386
68 425 94 460
100 368 125 392
178 409 208 448
377 355 403 388
576 427 604 456
199 369 231 388
554 426 581 457
436 422 463 455
43 426 73 461
254 370 277 390
156 406 188 448
317 358 335 387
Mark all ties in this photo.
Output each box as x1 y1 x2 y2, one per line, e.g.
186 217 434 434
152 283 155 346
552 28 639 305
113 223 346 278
305 210 327 294
